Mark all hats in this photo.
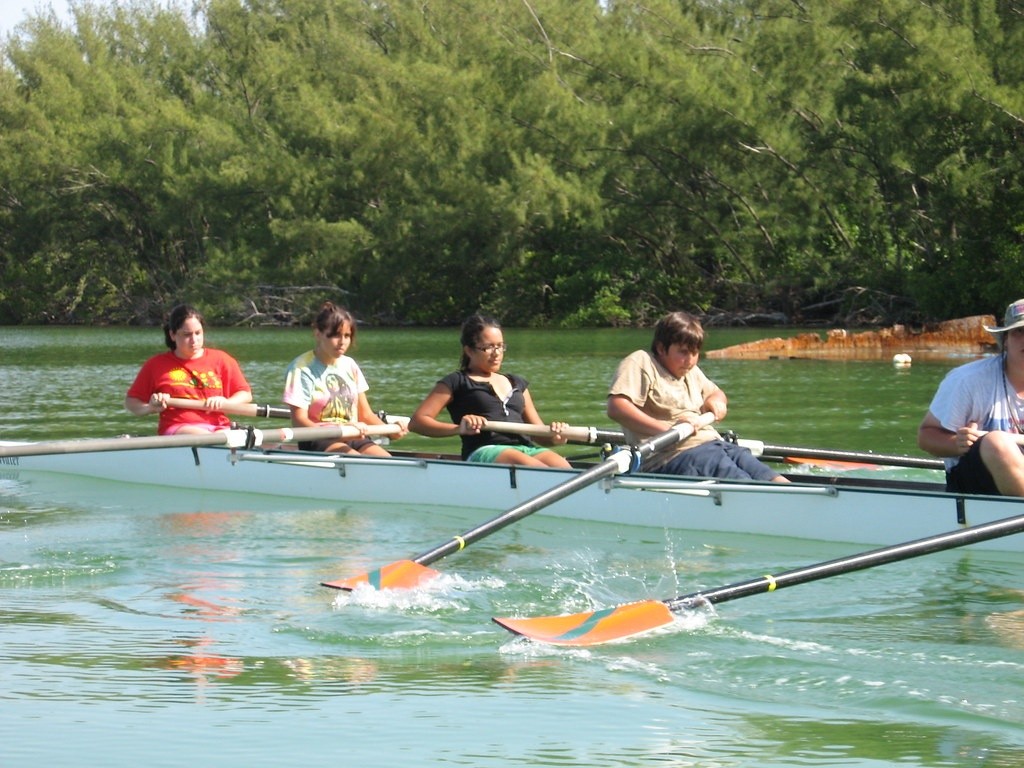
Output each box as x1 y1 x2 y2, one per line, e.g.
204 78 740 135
982 299 1024 342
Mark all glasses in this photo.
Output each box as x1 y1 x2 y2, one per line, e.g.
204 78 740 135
472 344 507 353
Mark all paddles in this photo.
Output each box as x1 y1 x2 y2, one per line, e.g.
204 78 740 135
490 513 1024 647
466 419 945 471
0 423 401 457
319 410 718 592
151 395 880 471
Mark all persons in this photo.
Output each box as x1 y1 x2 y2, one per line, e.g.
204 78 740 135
124 305 252 435
607 311 790 483
409 315 574 467
284 301 408 456
917 299 1024 497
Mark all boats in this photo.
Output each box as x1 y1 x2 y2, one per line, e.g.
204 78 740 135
0 436 1024 556
